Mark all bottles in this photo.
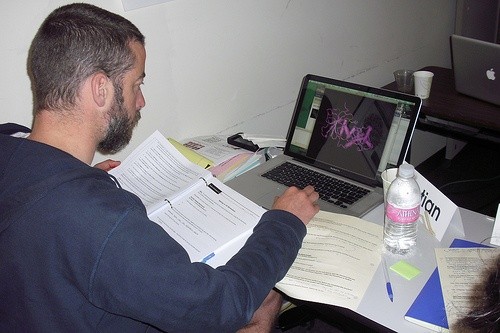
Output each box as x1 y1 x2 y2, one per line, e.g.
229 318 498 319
382 165 421 255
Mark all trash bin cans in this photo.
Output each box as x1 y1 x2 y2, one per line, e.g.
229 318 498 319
382 258 393 302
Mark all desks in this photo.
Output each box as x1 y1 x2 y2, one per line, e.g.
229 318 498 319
108 66 500 333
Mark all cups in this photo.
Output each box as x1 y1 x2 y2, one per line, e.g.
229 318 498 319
380 168 398 206
413 71 434 98
393 69 414 93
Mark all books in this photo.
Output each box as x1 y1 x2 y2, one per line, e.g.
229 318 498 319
109 129 268 264
168 133 289 183
270 203 391 313
403 238 500 333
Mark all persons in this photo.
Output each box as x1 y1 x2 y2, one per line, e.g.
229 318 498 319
0 3 322 333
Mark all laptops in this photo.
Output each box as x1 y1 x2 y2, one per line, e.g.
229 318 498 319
448 34 500 106
222 73 422 219
